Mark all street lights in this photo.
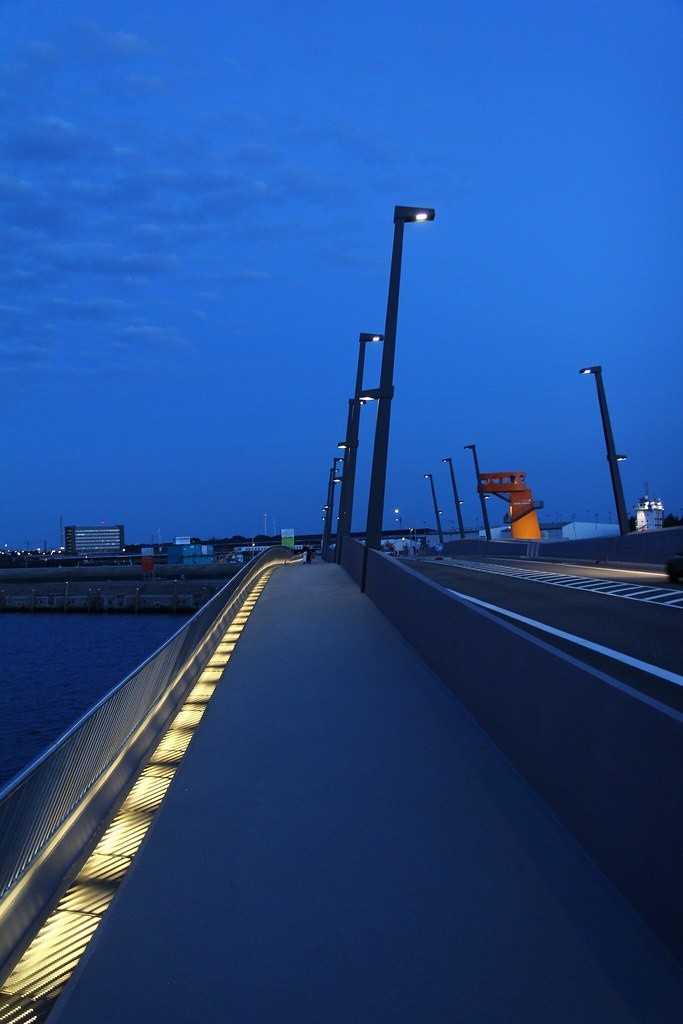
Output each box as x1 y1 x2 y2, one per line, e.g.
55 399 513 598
393 508 418 556
264 514 268 536
336 398 366 537
338 332 386 537
424 473 444 544
578 365 632 536
321 457 344 560
357 203 436 549
441 457 465 539
464 444 492 540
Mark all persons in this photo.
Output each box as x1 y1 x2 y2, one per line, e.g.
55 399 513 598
302 545 311 562
